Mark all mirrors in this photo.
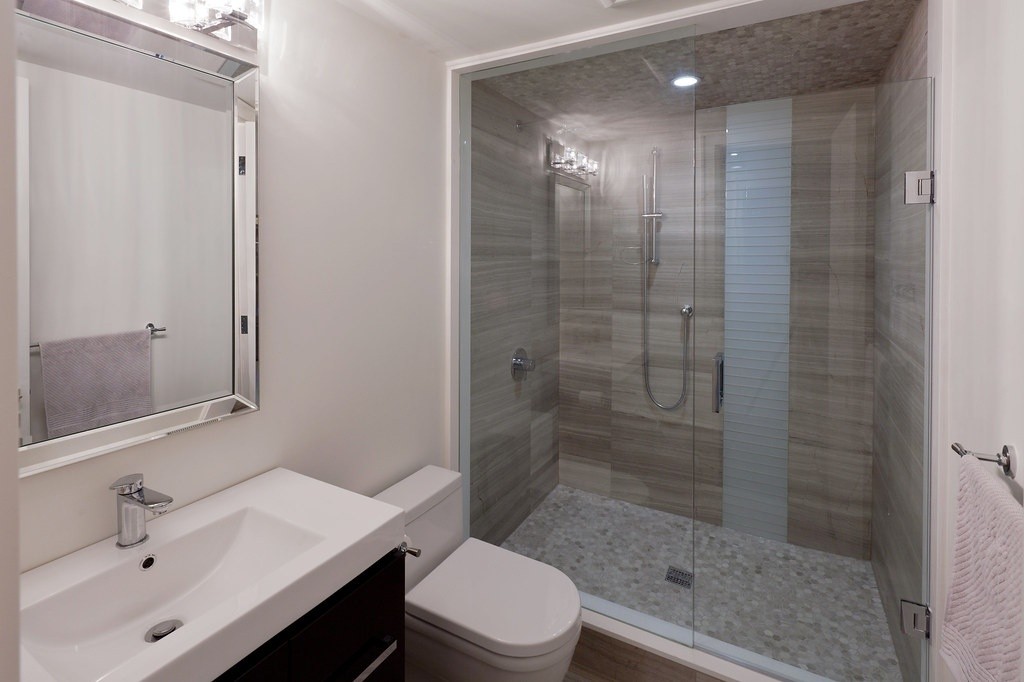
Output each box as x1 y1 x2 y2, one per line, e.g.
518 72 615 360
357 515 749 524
15 0 261 477
547 173 592 327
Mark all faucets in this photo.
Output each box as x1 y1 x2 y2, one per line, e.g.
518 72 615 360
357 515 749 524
109 472 173 550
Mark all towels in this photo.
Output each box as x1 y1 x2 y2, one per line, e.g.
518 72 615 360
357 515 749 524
38 328 152 440
935 454 1024 682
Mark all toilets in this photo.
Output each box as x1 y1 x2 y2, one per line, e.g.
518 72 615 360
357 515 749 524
369 462 583 682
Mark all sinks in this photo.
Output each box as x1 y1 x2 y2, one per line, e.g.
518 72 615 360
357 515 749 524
18 465 404 682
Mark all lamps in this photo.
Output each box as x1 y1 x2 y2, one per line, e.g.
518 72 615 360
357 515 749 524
549 141 600 180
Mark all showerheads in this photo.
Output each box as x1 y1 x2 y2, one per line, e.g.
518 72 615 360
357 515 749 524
650 218 659 267
